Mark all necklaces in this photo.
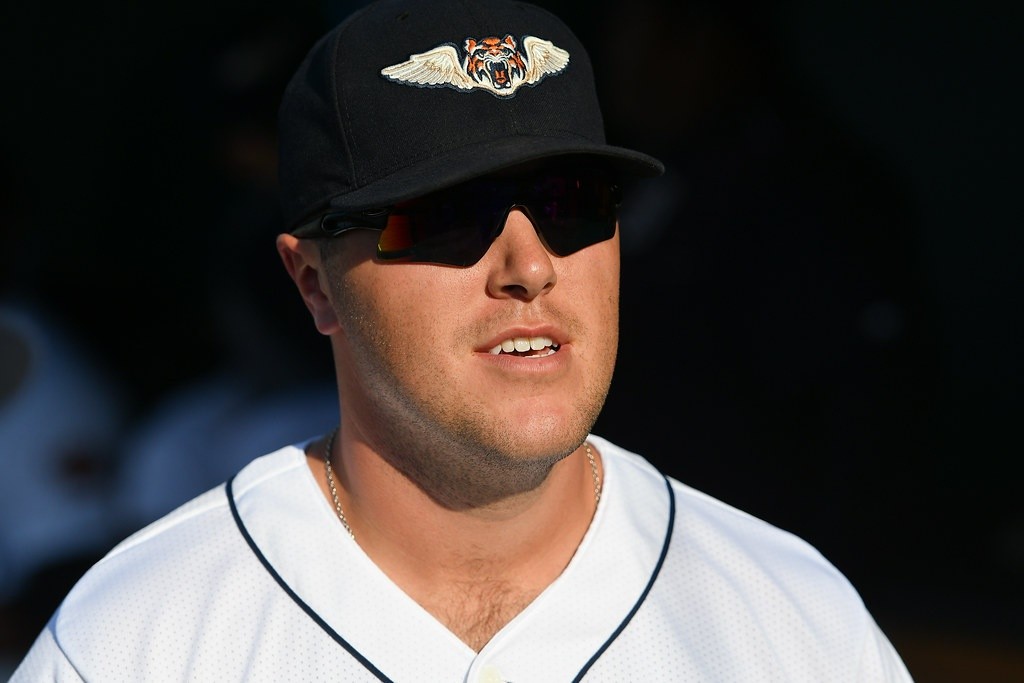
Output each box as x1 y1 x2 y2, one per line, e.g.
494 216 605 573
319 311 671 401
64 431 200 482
325 428 602 538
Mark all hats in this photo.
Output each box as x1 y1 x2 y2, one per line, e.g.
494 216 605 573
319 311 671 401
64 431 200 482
279 0 667 240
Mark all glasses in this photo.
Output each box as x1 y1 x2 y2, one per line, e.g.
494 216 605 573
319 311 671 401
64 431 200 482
290 156 622 268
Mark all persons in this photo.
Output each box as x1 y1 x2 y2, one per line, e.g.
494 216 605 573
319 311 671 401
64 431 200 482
9 0 914 682
